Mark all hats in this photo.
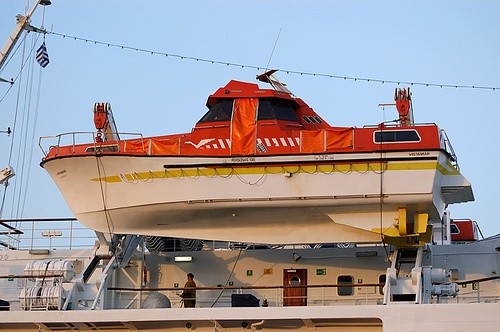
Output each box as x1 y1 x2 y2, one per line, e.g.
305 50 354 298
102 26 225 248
187 273 194 277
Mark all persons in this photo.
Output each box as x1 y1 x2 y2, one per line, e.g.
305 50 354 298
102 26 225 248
178 272 196 307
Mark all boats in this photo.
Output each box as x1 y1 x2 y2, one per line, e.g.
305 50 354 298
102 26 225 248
0 1 499 332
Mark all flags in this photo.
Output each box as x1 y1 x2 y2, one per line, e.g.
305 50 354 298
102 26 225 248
36 29 51 69
